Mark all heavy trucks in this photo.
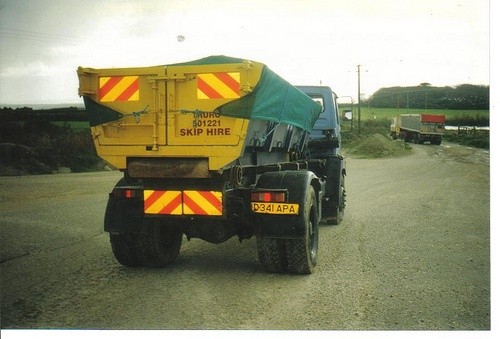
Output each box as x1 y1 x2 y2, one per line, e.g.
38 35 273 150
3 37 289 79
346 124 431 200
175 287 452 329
75 54 348 276
390 114 446 145
